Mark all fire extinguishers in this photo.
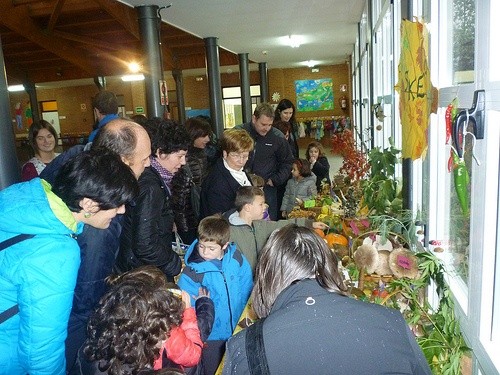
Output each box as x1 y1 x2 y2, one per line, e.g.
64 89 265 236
339 94 346 109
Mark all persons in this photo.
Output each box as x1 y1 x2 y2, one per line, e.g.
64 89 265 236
83 90 123 146
200 129 255 226
176 214 253 375
38 119 152 375
221 186 330 281
79 266 190 375
0 143 140 375
20 120 62 181
112 117 189 282
127 96 332 222
161 284 215 372
111 264 203 371
221 221 437 375
